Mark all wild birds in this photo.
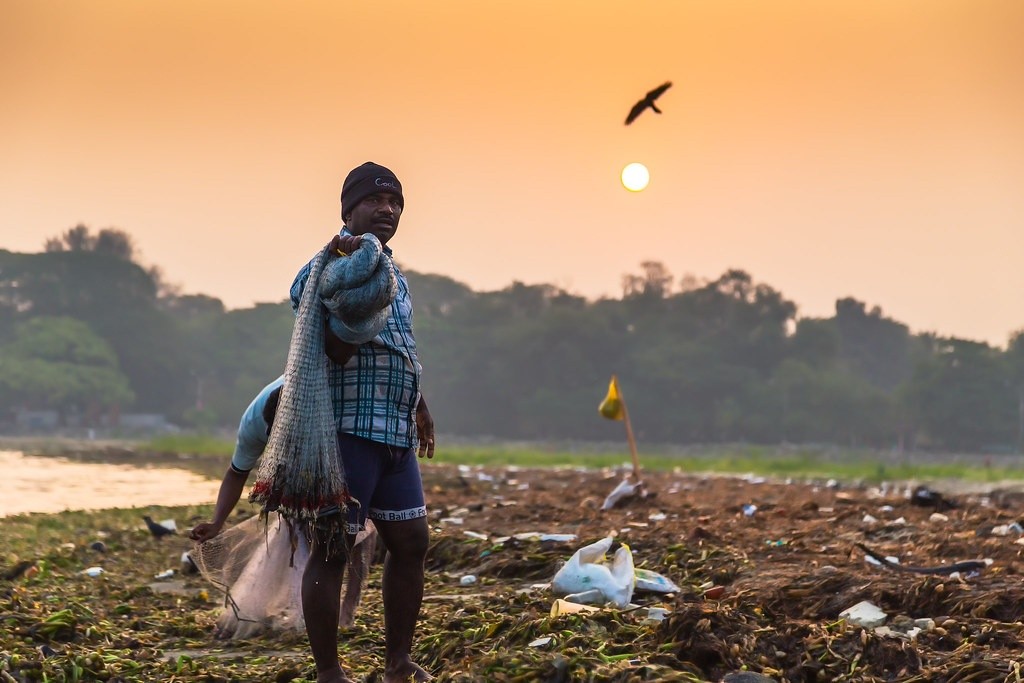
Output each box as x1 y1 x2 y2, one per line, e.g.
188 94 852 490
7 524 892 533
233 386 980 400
625 82 673 127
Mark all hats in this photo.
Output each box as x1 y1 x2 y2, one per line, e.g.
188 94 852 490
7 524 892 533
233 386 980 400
341 162 404 221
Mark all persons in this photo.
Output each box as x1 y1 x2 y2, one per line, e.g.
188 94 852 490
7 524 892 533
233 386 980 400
289 161 439 683
189 371 375 628
